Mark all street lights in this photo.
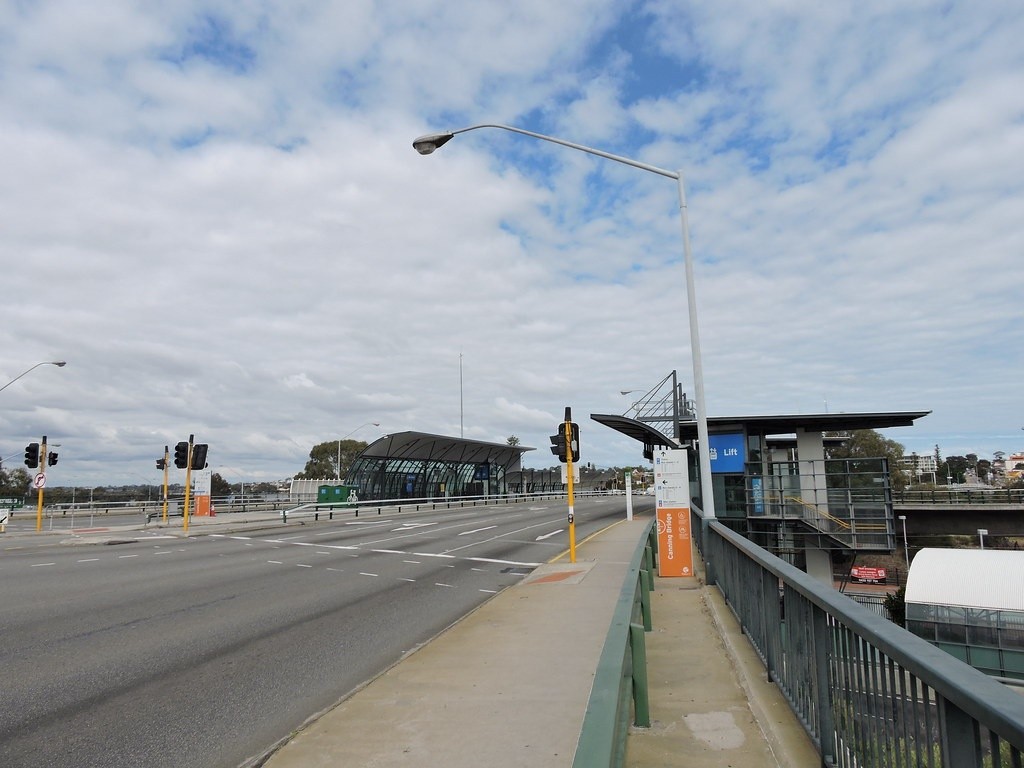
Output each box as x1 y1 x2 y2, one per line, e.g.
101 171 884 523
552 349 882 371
413 122 716 518
898 516 910 571
337 422 381 478
977 528 989 550
219 463 244 502
622 389 699 483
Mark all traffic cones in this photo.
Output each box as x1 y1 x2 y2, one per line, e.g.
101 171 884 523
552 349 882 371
210 502 216 517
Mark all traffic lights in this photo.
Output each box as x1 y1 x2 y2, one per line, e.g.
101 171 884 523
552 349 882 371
156 458 164 470
48 452 58 466
550 431 567 456
174 442 189 468
25 443 38 468
